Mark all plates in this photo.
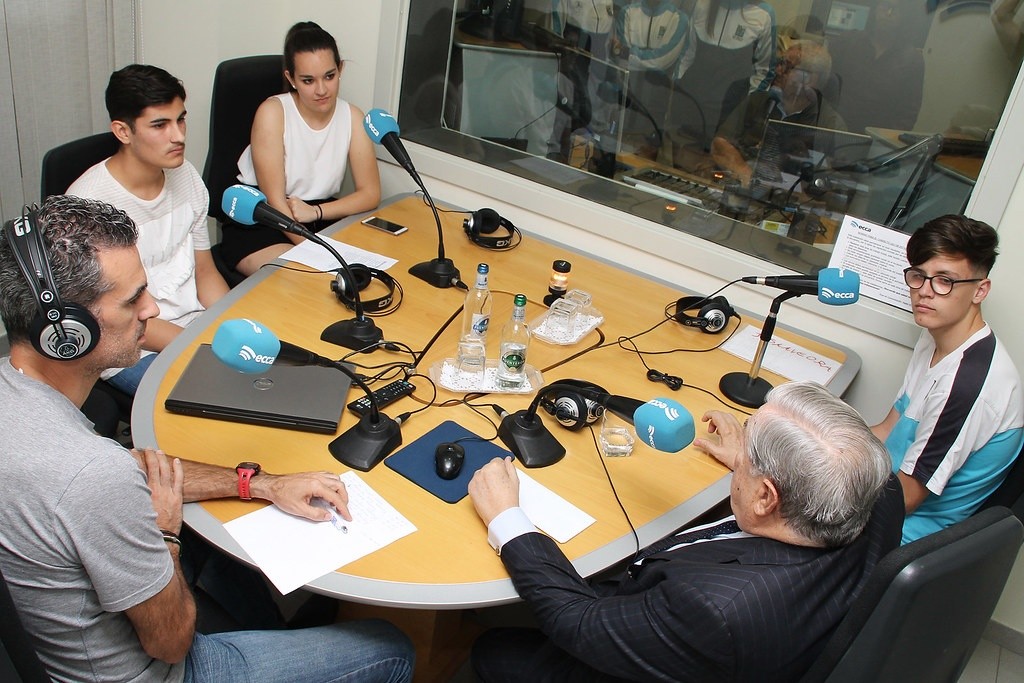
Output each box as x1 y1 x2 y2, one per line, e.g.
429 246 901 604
428 358 544 394
529 307 606 345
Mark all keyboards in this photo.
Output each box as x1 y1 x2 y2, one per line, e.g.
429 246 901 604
898 132 990 160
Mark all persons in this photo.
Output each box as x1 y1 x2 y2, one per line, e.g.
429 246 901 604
829 0 925 133
222 21 380 278
468 381 905 683
0 195 416 683
869 214 1024 546
64 64 230 396
711 39 849 189
545 0 776 169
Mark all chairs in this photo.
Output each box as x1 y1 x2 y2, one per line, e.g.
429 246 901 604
799 443 1023 683
0 563 52 683
40 130 132 427
199 55 289 288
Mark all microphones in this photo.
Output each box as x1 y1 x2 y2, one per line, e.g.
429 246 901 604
742 268 860 305
211 319 403 472
222 185 385 354
363 108 461 289
763 86 784 124
498 384 696 468
596 66 712 163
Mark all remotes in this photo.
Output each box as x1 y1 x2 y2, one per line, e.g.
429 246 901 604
347 379 417 419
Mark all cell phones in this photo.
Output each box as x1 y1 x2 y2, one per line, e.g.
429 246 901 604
361 217 408 236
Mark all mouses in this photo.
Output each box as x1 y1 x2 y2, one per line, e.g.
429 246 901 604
435 441 465 481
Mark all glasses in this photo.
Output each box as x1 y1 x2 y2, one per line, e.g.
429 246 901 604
904 267 984 296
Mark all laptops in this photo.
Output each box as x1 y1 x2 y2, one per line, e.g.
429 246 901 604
164 343 356 436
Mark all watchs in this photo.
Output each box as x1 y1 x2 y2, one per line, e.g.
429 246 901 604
236 462 261 500
614 44 623 56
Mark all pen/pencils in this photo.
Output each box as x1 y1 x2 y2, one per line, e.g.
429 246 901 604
318 496 348 535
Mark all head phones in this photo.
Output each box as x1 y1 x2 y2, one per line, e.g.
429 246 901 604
664 295 734 334
4 202 101 360
330 263 404 317
463 208 522 252
540 379 610 432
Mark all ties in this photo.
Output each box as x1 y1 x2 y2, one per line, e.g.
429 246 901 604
631 520 743 562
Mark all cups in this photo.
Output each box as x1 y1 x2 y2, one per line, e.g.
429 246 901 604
544 298 578 340
452 339 485 387
565 288 592 329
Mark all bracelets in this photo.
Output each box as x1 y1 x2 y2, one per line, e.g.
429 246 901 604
313 204 322 220
160 530 182 558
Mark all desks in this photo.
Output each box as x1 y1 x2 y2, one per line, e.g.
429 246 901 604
863 126 989 216
451 15 561 147
130 191 864 683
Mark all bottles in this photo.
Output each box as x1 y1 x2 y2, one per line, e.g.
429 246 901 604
462 263 492 344
495 294 531 391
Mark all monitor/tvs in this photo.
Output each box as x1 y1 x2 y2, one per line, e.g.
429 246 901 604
545 48 629 175
744 119 872 236
